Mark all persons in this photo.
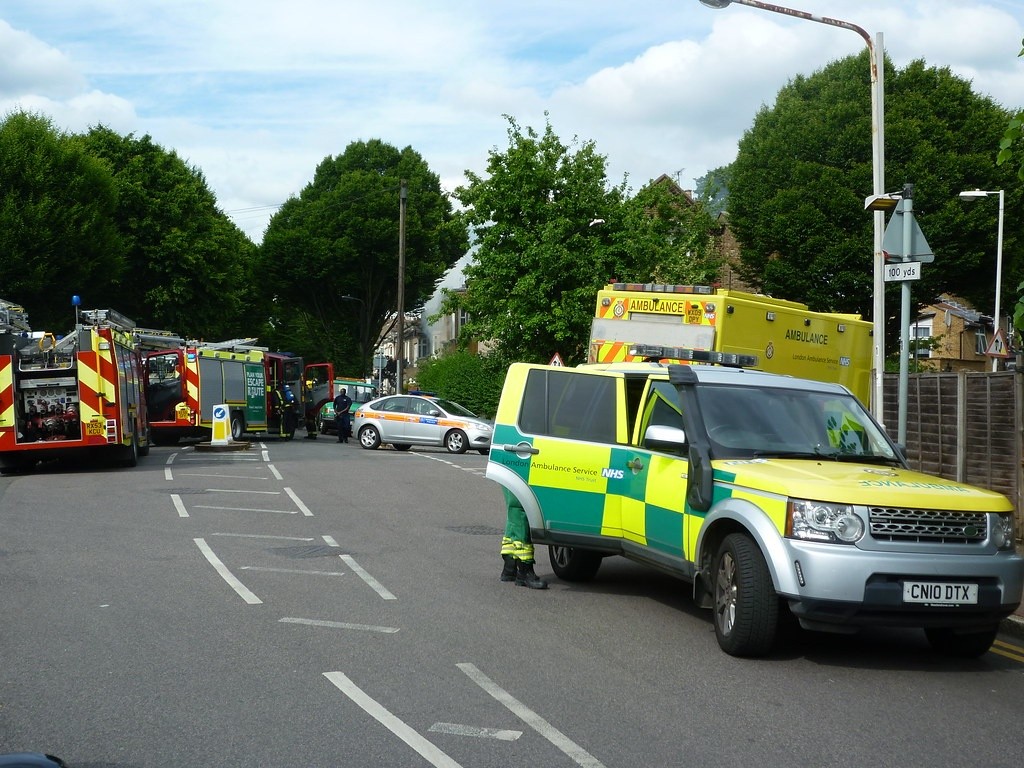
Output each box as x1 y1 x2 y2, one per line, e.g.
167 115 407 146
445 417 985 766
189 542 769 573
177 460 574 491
333 387 352 443
500 482 548 589
303 390 318 440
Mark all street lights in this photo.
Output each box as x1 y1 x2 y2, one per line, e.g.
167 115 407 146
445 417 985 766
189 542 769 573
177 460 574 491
342 296 366 345
959 189 1004 378
862 182 913 458
700 0 887 439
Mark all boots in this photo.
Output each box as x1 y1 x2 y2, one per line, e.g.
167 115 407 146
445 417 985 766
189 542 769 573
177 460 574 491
498 554 517 581
516 559 548 588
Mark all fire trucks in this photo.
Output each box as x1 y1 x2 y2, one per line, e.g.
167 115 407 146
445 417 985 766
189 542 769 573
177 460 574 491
0 295 150 467
145 338 334 446
132 327 184 365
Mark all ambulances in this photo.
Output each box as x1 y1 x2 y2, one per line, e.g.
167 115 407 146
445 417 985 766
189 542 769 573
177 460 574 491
585 280 875 419
483 341 1023 665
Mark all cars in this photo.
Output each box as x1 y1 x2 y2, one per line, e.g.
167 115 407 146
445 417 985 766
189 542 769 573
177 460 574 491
351 390 494 454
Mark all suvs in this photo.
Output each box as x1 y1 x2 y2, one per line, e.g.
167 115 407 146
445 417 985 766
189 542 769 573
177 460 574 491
316 376 377 434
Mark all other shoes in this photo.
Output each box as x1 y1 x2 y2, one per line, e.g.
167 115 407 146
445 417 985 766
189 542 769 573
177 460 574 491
345 440 348 443
336 439 343 443
304 435 316 440
